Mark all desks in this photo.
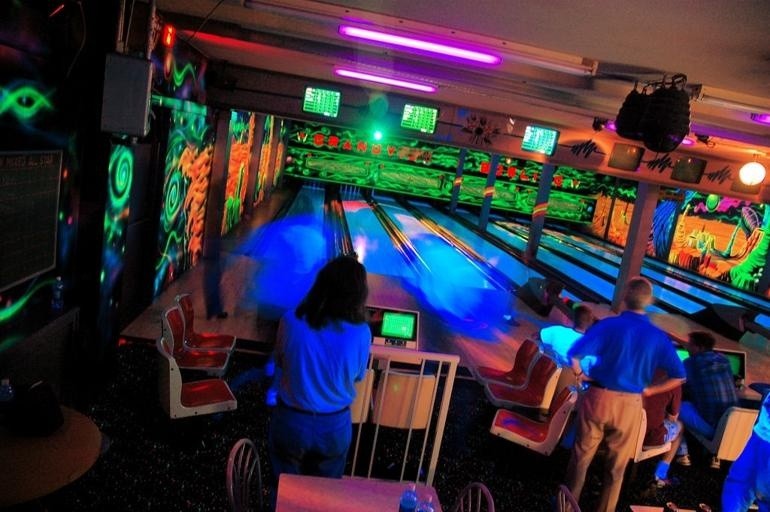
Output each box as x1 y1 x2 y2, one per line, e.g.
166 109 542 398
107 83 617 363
276 472 444 512
0 407 103 512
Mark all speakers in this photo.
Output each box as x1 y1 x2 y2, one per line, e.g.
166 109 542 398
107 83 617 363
99 53 154 136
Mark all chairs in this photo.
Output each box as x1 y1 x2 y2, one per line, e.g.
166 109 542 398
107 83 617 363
692 406 760 493
471 337 541 414
363 368 436 479
491 385 578 485
484 353 562 422
350 369 375 425
226 438 266 512
163 302 229 377
154 338 238 488
450 480 495 512
349 345 462 487
175 292 236 352
624 408 671 500
556 486 582 512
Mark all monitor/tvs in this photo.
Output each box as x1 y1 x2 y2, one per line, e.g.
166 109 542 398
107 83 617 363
521 123 560 156
727 355 742 377
677 349 691 365
400 102 440 135
608 140 645 173
380 311 418 342
670 152 707 185
301 85 341 120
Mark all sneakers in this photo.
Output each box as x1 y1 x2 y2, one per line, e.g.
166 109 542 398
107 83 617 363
677 454 694 468
710 456 721 469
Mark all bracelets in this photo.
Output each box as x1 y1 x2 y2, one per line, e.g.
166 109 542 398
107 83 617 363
574 370 584 376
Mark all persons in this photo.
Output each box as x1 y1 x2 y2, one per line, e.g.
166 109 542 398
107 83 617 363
267 255 372 512
721 387 770 512
677 331 741 469
531 306 597 376
642 368 684 489
563 276 687 512
